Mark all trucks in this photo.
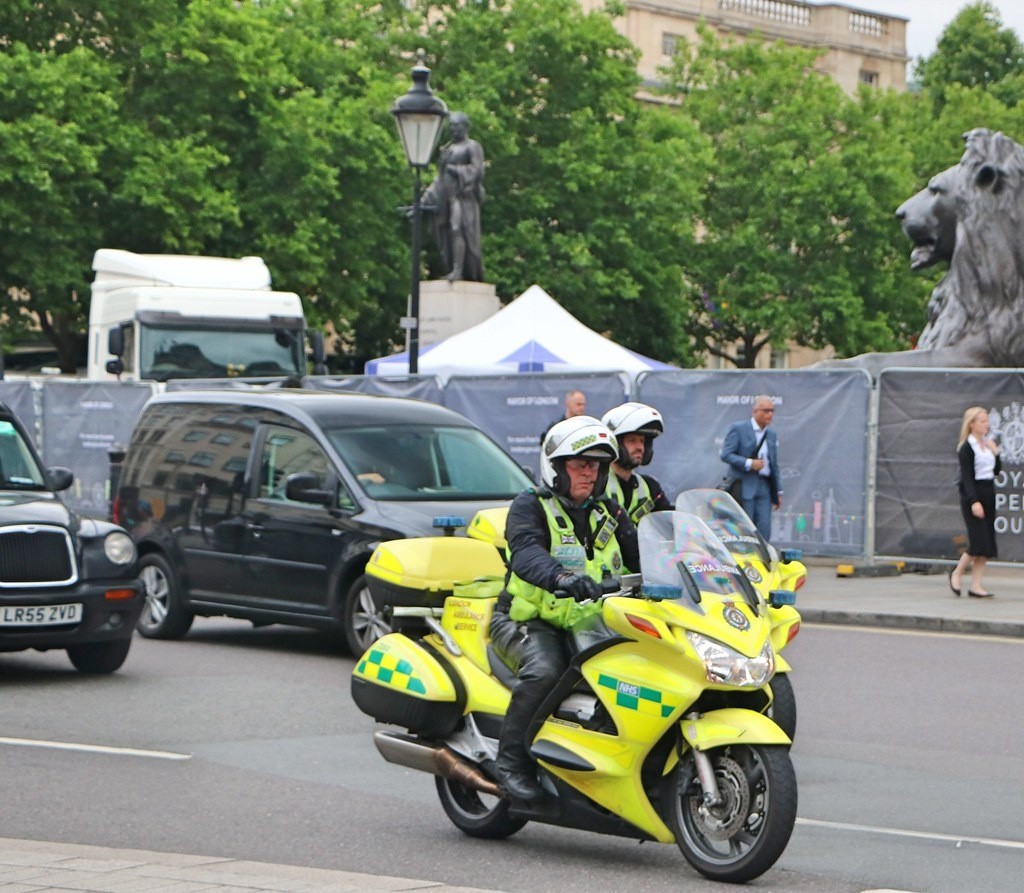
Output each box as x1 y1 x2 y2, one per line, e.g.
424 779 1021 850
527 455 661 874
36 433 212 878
87 249 325 383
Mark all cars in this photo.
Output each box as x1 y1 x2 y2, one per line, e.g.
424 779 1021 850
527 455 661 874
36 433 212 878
111 391 540 661
0 401 146 674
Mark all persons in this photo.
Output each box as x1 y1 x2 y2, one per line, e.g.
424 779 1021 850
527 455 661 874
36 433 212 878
601 402 678 582
304 450 384 506
396 110 486 280
539 388 586 446
948 407 1002 599
715 395 784 544
489 415 642 802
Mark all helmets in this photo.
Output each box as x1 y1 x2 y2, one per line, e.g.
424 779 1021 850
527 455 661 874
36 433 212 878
602 402 664 470
541 415 620 497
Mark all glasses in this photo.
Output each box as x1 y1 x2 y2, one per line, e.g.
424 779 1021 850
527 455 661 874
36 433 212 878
758 409 774 413
563 456 601 471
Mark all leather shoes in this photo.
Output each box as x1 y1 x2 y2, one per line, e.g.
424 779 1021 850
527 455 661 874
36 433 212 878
495 763 545 801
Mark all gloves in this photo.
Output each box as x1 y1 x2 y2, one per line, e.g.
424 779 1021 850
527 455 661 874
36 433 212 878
555 570 602 603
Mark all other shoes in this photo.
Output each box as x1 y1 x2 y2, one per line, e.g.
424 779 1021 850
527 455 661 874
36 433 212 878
949 570 961 596
968 589 994 598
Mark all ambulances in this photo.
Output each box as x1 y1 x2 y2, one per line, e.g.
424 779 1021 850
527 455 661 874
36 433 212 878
350 488 807 884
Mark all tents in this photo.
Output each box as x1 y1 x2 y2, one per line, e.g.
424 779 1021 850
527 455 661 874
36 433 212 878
363 283 682 401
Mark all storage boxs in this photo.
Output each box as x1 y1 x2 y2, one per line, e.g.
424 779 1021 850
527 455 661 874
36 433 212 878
366 538 508 633
466 507 510 563
351 632 468 744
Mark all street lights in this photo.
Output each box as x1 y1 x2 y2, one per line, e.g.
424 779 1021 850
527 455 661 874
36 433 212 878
391 47 450 373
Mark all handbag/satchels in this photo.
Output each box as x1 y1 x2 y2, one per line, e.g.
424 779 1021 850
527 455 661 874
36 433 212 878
718 476 743 509
